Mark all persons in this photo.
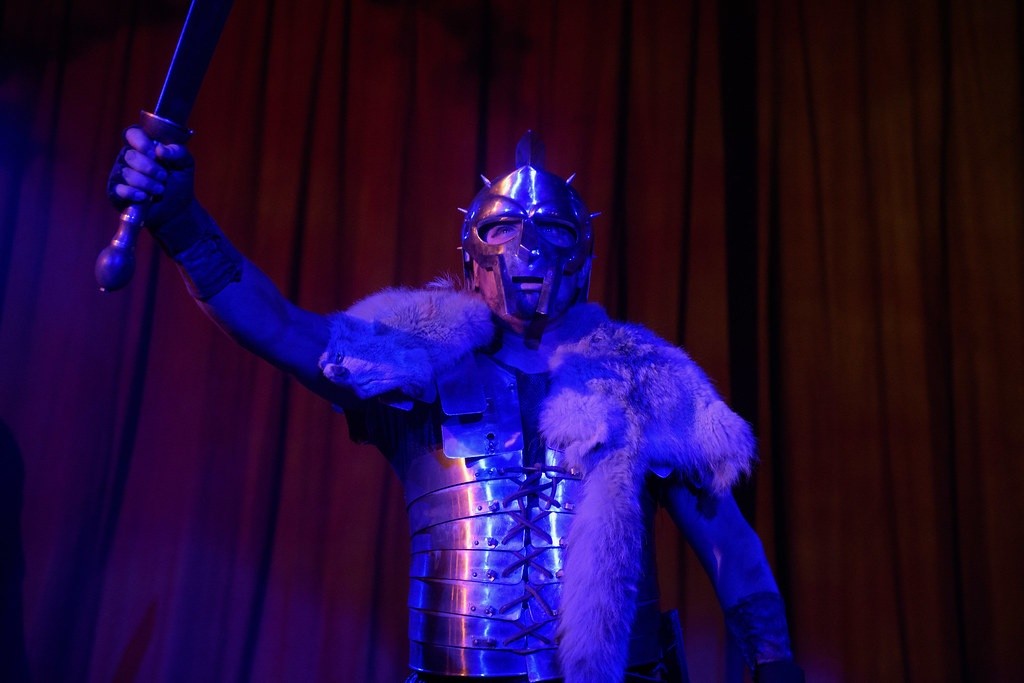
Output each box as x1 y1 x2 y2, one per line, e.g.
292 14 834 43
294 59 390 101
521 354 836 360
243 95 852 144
103 107 810 683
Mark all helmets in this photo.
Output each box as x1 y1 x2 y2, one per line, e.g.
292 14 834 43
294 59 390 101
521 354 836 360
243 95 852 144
455 129 603 320
